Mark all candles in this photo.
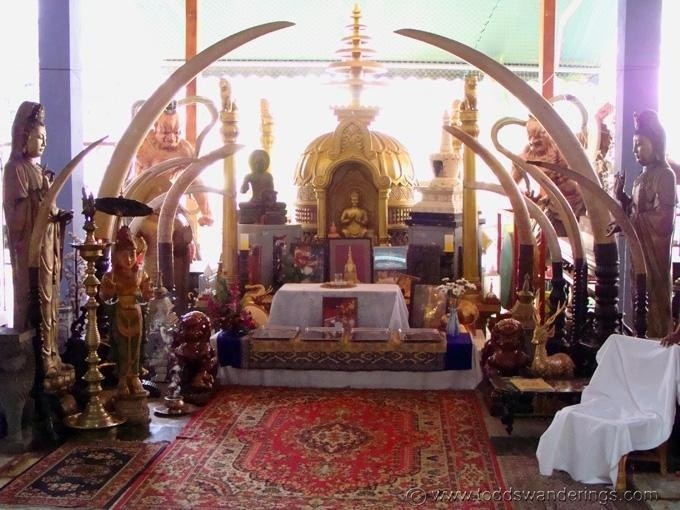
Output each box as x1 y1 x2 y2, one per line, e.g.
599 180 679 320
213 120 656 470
239 233 249 251
446 235 453 251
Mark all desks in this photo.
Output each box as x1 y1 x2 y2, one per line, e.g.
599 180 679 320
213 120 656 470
266 283 411 334
490 374 592 434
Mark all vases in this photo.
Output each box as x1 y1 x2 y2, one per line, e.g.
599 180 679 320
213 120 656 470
446 303 461 337
224 329 242 336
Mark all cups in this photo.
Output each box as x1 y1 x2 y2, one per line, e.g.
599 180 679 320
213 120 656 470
334 272 342 285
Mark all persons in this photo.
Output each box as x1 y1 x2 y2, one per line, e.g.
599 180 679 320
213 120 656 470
505 108 595 239
602 110 676 341
98 227 155 398
658 324 679 348
237 150 288 211
3 101 73 387
341 188 370 239
134 106 214 288
274 238 304 283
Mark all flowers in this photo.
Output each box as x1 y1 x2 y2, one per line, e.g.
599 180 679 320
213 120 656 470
206 271 258 333
435 276 477 309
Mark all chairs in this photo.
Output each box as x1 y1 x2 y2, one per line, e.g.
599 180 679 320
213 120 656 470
535 333 679 495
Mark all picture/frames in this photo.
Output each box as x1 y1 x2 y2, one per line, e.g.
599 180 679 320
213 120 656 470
328 238 374 284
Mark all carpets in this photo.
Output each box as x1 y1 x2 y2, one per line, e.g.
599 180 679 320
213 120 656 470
0 438 169 510
108 384 515 510
489 435 653 510
